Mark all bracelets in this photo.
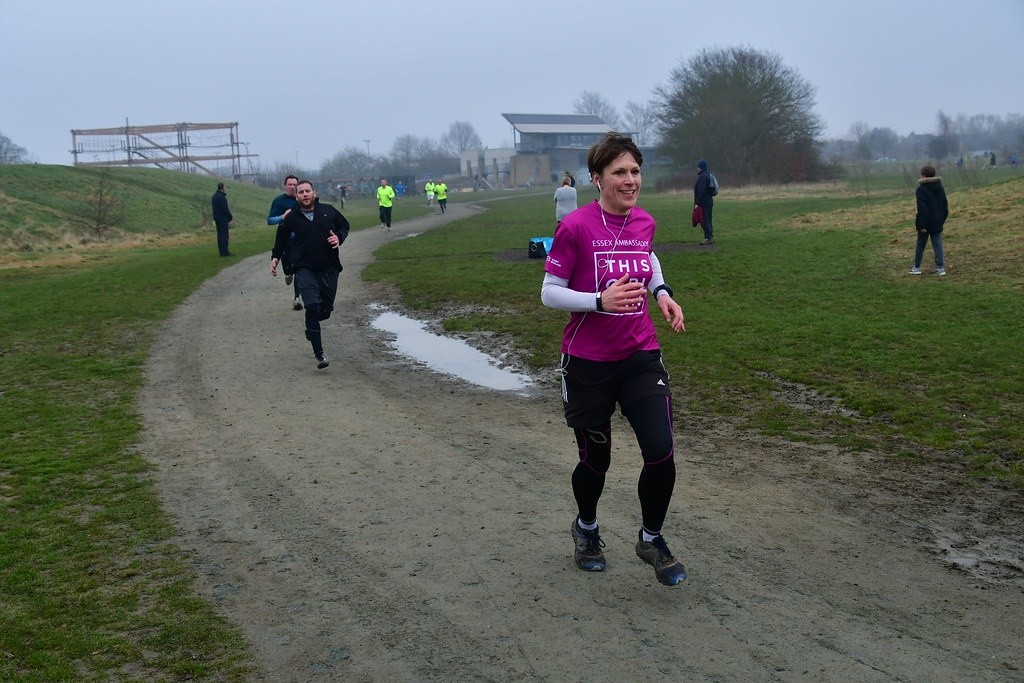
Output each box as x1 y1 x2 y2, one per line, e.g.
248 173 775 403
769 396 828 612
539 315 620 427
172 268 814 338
596 292 603 311
653 284 673 302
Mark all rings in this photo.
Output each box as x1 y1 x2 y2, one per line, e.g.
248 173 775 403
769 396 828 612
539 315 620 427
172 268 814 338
336 241 337 243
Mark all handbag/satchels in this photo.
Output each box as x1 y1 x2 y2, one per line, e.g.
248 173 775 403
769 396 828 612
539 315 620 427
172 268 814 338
709 172 719 196
692 206 703 227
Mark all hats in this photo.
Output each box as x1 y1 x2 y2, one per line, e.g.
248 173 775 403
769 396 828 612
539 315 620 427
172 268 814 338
697 158 707 169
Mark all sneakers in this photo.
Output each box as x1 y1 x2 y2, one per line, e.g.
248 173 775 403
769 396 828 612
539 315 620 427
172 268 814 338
635 529 687 585
571 515 607 570
907 266 922 274
929 267 947 275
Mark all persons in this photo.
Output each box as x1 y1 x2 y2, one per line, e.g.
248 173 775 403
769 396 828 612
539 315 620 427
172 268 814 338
395 180 405 200
425 179 436 206
211 183 235 257
434 179 448 214
565 171 575 187
553 176 578 223
267 175 304 311
339 182 347 202
694 159 714 244
988 152 997 170
541 131 688 586
1010 152 1018 168
907 166 949 275
376 179 395 231
270 180 350 368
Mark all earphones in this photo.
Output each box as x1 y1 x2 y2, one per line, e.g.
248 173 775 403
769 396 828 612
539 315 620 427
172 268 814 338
597 180 602 190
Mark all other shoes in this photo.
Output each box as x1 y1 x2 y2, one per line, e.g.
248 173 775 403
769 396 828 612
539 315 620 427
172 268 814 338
292 297 303 309
387 226 391 230
700 238 713 244
314 351 329 369
381 222 386 228
